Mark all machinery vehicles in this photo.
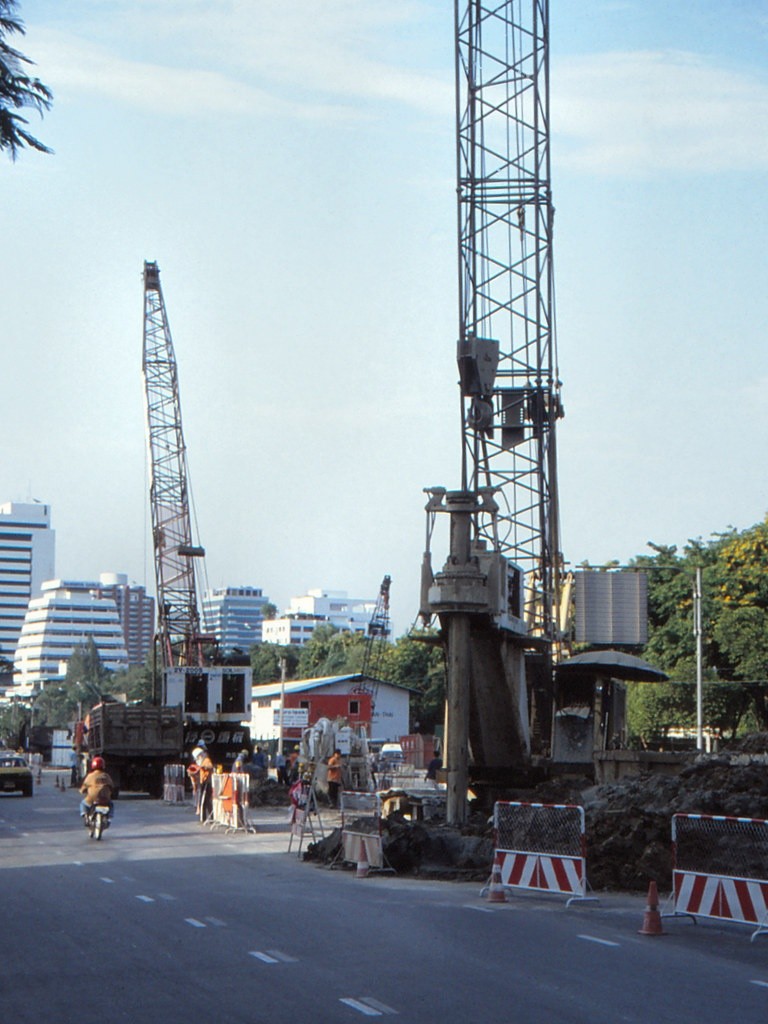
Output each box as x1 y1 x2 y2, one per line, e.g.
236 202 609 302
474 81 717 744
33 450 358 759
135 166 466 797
138 256 254 760
409 1 671 838
301 572 391 779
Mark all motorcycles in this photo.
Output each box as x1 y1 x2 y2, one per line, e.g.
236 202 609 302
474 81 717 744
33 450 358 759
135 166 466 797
80 788 112 840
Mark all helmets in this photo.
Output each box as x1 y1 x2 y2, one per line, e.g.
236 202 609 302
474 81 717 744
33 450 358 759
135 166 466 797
91 757 106 770
294 745 300 750
192 747 203 760
242 750 249 757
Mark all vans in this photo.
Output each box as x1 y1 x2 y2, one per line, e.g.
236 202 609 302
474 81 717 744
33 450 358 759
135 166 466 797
381 742 407 769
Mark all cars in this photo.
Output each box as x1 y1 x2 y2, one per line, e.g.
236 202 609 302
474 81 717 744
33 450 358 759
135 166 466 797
0 753 33 797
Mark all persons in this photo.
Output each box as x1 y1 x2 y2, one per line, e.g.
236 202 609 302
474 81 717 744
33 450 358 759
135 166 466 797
253 744 301 787
327 749 344 809
79 757 115 828
424 750 442 783
192 740 250 823
69 745 85 786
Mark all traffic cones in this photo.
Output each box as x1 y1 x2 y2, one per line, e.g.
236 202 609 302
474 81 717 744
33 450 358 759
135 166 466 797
488 858 507 903
36 770 66 792
638 882 669 936
187 764 199 794
354 840 375 878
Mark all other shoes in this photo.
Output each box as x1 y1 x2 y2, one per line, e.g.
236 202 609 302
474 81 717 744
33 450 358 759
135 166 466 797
85 821 89 826
102 820 110 825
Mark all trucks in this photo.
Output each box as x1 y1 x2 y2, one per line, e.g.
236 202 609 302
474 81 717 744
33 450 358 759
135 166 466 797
77 702 183 799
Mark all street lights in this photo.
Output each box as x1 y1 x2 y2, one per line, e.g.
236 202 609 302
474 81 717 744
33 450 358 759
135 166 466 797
246 624 288 781
